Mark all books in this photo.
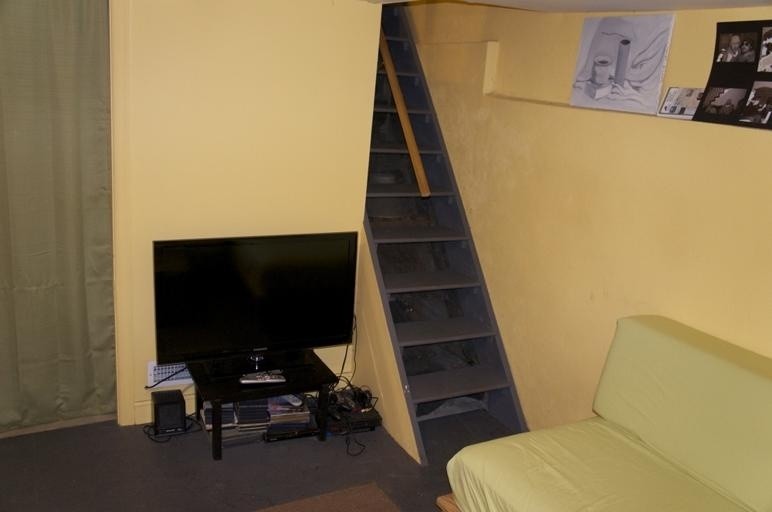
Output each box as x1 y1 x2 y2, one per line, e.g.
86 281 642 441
197 391 312 446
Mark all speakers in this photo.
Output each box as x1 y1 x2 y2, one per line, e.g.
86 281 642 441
150 390 187 437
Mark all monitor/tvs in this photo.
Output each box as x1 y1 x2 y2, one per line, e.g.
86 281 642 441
150 231 359 367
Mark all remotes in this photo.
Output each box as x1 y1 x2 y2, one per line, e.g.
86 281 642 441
281 394 302 407
239 373 286 384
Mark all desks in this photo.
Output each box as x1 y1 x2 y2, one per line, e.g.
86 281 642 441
184 348 339 460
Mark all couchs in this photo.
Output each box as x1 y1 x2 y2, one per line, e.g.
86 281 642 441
436 316 772 512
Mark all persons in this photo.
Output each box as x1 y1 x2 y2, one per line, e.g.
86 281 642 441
703 31 772 126
